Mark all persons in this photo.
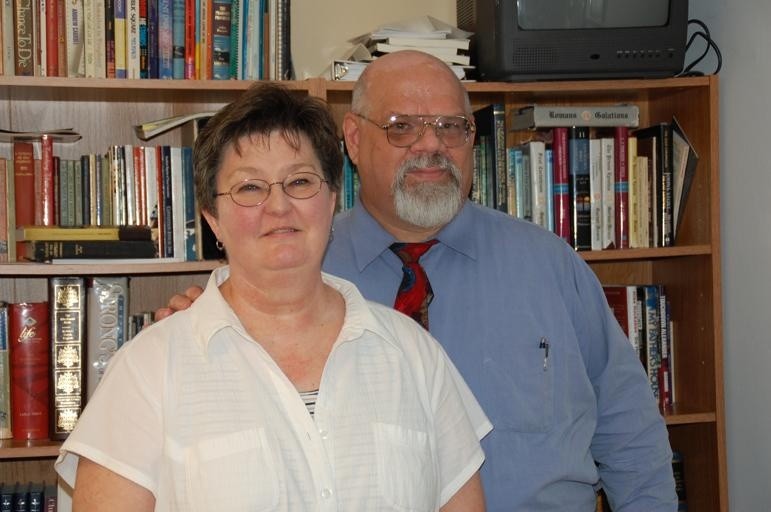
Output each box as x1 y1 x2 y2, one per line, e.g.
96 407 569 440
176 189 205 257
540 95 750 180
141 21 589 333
55 77 495 512
139 45 681 511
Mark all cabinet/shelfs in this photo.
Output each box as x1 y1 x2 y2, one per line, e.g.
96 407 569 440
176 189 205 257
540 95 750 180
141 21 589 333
0 79 728 512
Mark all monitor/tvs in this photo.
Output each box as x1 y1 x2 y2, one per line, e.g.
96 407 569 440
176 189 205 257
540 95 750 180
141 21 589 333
457 0 688 82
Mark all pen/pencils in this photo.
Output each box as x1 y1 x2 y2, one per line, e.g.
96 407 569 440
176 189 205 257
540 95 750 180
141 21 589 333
539 336 549 372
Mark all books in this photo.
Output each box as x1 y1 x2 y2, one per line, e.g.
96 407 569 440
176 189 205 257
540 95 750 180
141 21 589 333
319 14 478 84
336 139 359 213
600 283 676 415
672 454 688 512
468 100 701 252
1 0 295 81
0 276 155 441
1 473 73 512
0 110 229 264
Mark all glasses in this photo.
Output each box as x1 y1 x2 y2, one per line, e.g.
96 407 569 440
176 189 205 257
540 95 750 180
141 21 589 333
211 172 330 208
354 110 477 148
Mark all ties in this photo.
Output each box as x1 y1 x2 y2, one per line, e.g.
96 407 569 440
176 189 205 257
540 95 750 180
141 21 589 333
386 238 441 331
